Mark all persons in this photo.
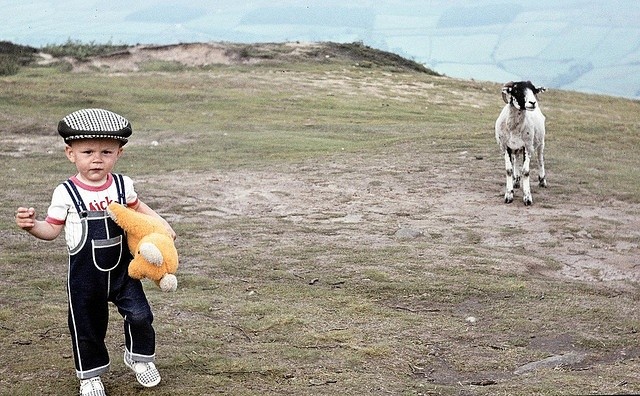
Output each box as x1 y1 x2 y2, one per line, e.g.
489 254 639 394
15 109 162 396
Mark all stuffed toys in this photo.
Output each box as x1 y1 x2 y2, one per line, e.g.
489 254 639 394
106 202 178 292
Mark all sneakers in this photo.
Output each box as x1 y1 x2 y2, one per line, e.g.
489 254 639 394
123 350 162 388
79 376 107 396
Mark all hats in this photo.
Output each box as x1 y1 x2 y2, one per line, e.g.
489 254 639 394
57 107 133 148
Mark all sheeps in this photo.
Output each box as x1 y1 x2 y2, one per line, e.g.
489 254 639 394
494 79 547 205
